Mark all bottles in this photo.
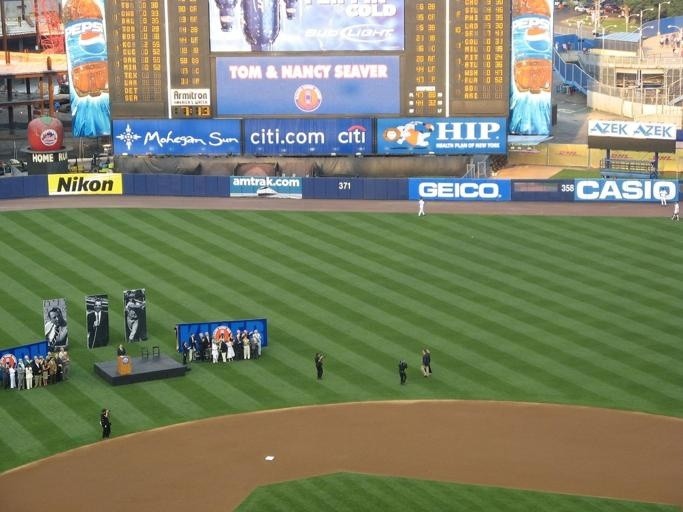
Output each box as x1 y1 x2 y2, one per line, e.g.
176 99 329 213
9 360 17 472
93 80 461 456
63 0 110 98
91 153 96 165
511 0 553 94
241 0 281 51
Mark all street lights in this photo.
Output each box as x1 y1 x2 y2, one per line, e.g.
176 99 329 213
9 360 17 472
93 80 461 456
575 2 683 60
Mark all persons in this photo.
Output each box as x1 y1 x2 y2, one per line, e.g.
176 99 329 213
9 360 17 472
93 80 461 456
314 352 323 380
657 188 667 207
100 408 111 440
182 329 262 365
398 359 406 385
117 344 127 356
417 196 426 216
125 293 145 343
86 299 107 348
421 348 430 377
44 307 68 351
670 201 679 221
2 347 69 390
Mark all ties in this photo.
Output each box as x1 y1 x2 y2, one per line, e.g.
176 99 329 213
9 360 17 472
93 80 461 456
50 326 59 347
